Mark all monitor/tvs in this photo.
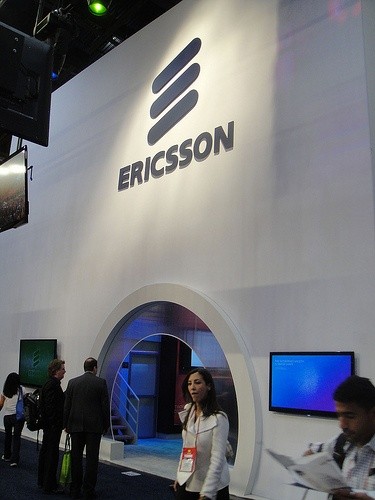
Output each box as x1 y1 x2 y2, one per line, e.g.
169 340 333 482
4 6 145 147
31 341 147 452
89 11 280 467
268 351 355 418
18 339 57 387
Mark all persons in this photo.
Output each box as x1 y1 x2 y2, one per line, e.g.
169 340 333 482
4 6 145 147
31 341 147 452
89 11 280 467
302 375 375 500
174 369 230 500
37 359 66 494
0 372 26 465
63 357 110 500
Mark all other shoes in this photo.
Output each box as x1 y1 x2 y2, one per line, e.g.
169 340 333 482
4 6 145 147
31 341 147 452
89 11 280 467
2 455 11 461
10 462 18 467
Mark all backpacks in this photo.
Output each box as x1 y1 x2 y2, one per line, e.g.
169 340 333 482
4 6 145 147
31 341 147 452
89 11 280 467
24 390 43 431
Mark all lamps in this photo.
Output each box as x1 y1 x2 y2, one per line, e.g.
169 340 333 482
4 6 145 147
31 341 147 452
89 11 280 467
51 45 66 79
86 0 112 15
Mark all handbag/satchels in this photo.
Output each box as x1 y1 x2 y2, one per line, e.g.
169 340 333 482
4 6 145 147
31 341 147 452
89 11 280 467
16 399 27 420
59 450 71 487
